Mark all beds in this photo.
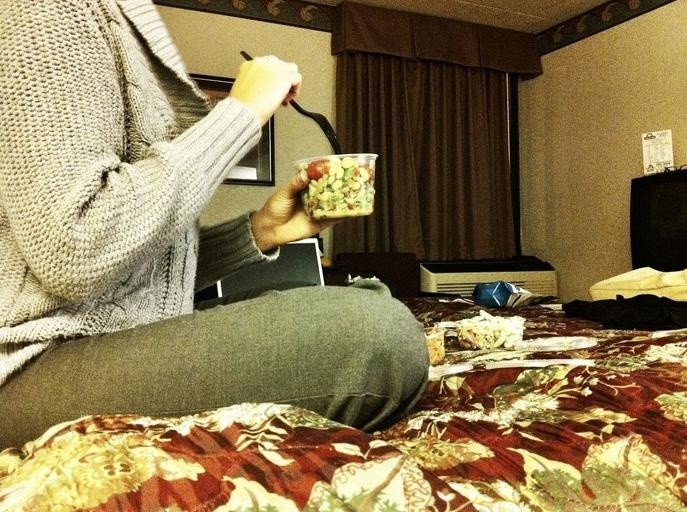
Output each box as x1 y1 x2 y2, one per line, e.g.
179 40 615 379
0 291 687 512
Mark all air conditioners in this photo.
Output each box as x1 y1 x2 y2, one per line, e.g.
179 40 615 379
422 259 560 304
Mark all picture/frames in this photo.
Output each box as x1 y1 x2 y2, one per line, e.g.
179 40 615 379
190 73 276 187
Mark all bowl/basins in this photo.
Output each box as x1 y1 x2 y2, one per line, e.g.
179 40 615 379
288 154 378 221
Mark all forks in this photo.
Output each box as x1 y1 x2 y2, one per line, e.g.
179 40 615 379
239 50 342 154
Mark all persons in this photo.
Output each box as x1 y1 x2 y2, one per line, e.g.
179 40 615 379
0 0 430 448
